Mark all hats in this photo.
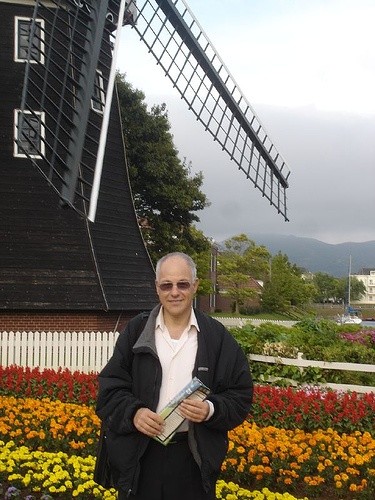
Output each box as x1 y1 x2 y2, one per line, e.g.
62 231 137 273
158 281 195 291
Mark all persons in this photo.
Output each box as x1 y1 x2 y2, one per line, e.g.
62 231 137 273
91 250 255 500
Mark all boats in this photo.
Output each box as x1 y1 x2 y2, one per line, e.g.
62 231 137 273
336 310 363 325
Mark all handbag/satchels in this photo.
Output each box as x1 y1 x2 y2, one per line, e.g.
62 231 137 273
94 418 143 496
